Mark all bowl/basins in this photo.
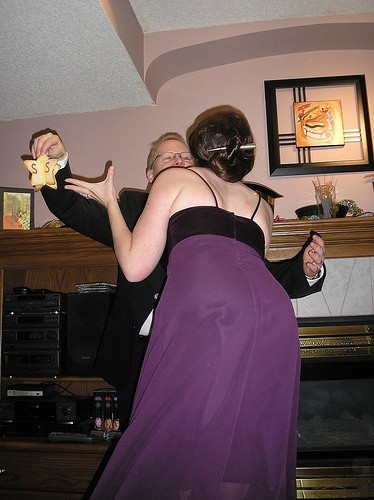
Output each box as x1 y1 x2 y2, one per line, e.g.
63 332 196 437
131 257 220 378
295 203 349 220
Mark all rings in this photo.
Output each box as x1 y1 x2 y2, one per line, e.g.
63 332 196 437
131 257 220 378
86 195 88 199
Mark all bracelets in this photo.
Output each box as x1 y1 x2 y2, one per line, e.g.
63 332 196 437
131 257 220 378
305 271 321 281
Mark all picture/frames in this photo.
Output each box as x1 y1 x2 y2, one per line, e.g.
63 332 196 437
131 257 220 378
263 74 374 178
1 186 35 230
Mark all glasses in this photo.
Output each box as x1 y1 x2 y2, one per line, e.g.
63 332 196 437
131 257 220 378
150 151 192 170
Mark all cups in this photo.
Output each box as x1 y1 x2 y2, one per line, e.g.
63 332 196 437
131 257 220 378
314 185 336 219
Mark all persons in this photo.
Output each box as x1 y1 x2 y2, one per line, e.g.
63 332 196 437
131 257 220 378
30 132 327 435
67 105 301 500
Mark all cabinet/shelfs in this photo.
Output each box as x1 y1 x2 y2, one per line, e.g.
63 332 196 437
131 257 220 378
0 216 116 494
